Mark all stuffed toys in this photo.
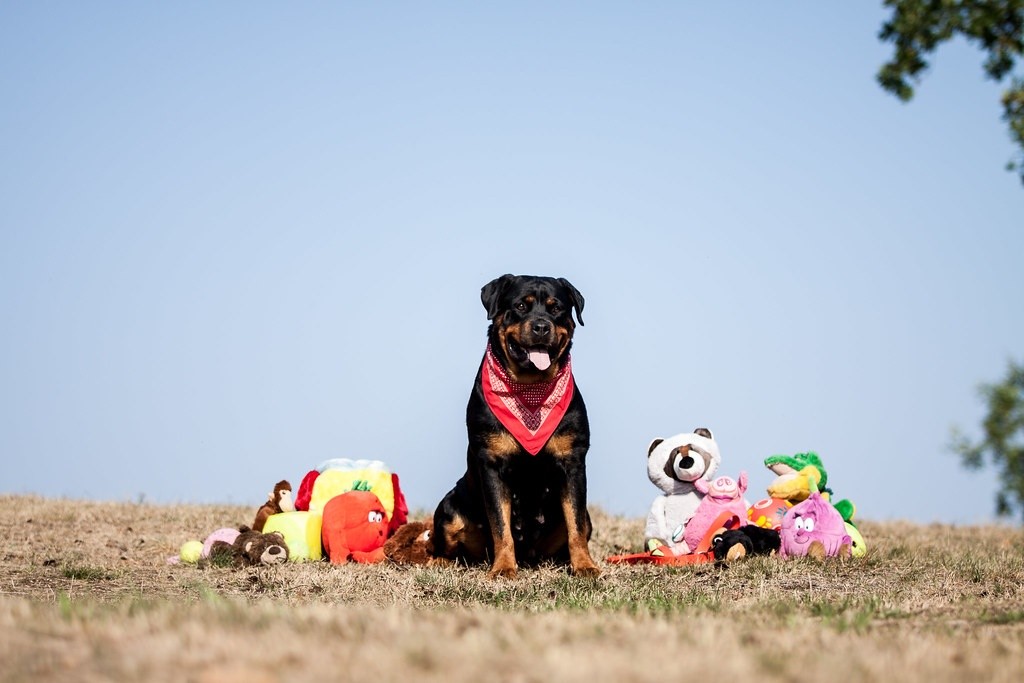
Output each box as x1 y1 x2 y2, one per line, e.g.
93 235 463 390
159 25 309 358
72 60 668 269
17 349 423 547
175 459 437 568
644 426 871 568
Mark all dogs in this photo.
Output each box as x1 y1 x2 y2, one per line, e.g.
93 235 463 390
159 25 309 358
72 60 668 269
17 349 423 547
431 274 605 583
